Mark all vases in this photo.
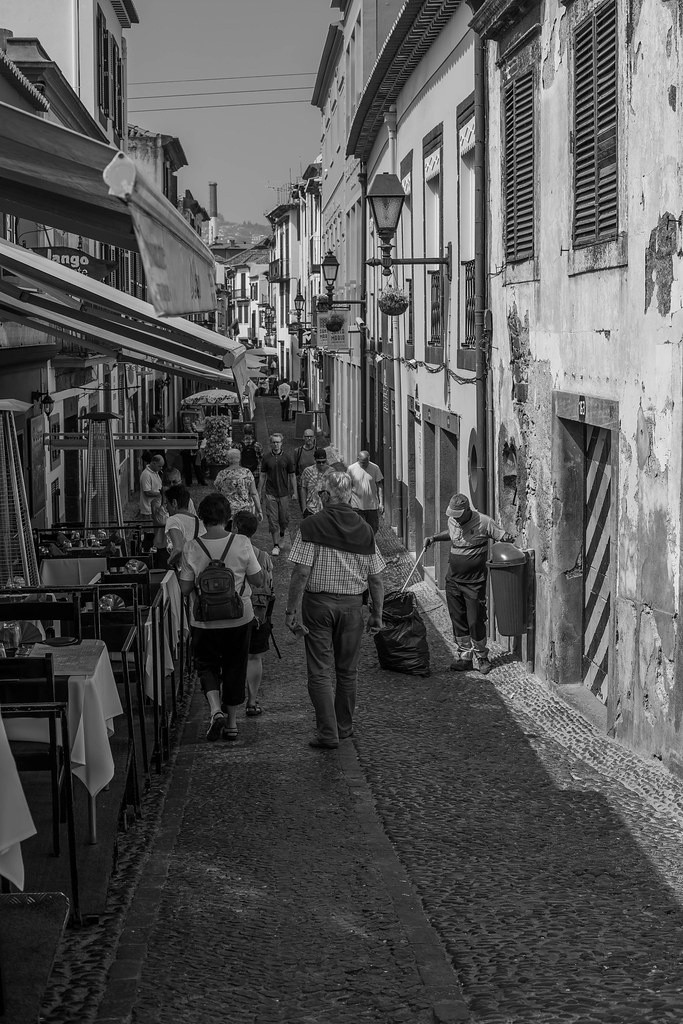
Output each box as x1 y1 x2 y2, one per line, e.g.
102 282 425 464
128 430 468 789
379 299 409 316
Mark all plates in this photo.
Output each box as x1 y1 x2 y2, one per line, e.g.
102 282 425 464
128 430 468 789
48 638 77 645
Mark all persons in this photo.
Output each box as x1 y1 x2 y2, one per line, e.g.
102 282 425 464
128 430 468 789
281 472 384 749
295 428 385 537
256 358 278 397
138 403 287 715
259 431 294 557
423 493 517 674
180 494 267 741
278 378 291 422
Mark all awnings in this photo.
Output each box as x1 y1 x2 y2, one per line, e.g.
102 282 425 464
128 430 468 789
0 103 219 320
0 242 260 419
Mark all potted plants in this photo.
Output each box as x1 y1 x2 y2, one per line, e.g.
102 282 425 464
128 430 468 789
326 313 345 332
203 416 232 480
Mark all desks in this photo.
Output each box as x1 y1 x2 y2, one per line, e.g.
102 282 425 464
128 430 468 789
38 558 108 587
0 640 125 846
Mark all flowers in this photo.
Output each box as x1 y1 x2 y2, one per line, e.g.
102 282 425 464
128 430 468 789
378 289 409 310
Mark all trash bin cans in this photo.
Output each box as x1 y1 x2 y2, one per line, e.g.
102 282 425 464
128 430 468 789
487 557 528 636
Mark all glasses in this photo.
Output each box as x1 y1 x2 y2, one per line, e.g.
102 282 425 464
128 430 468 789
316 461 326 463
317 490 330 497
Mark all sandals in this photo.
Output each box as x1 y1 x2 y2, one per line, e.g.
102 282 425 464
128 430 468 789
246 704 263 717
204 710 225 740
222 728 239 741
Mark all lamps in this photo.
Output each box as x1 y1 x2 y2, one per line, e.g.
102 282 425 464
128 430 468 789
363 172 452 282
294 292 311 326
31 389 55 420
318 249 368 313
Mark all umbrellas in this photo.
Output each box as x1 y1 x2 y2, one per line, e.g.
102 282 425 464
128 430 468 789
181 385 239 418
246 352 269 381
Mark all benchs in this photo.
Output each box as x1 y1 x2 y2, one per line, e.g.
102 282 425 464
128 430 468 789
0 521 195 930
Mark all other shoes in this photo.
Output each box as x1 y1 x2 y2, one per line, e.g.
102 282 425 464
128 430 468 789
272 546 281 556
477 657 491 674
308 739 324 748
339 727 354 739
451 659 473 670
279 540 286 548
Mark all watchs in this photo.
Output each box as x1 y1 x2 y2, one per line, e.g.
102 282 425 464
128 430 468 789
284 606 297 615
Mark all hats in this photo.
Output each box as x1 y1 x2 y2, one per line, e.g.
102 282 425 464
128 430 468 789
445 493 469 519
314 448 327 458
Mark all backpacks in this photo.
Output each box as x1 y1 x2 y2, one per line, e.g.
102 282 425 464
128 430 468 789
238 440 258 471
249 549 270 626
192 533 246 621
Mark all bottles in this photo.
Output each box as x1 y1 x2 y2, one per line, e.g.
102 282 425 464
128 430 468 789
79 539 88 547
0 643 6 658
